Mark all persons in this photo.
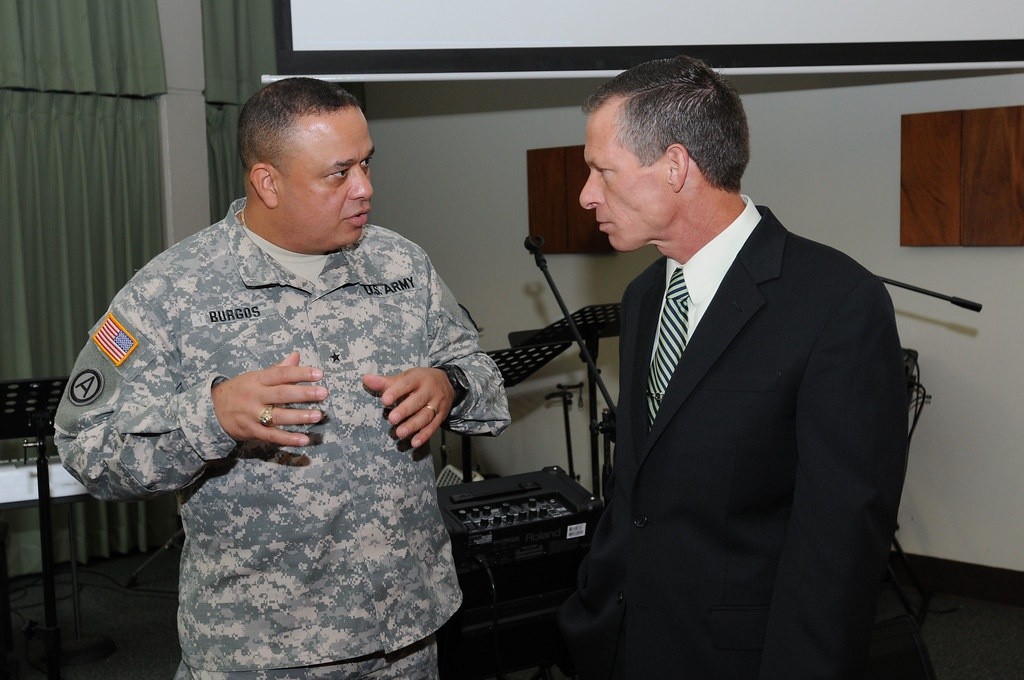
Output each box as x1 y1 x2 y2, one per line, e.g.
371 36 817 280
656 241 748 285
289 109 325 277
539 54 910 680
51 74 513 679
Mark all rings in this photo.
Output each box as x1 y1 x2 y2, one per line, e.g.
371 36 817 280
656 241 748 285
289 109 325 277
425 405 436 418
256 406 274 427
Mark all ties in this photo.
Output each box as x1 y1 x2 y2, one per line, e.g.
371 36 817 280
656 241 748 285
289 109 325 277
647 268 689 428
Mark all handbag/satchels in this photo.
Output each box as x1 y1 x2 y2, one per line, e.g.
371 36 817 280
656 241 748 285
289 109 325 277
866 614 937 679
